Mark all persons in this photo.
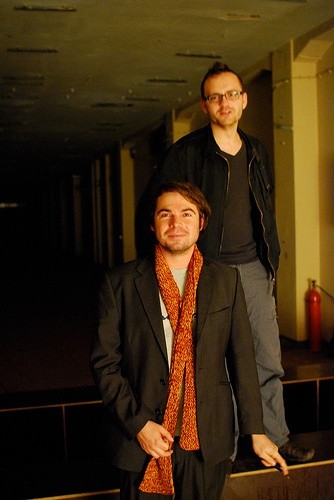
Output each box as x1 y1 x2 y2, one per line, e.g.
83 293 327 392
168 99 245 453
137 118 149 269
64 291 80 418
90 179 289 500
162 62 316 460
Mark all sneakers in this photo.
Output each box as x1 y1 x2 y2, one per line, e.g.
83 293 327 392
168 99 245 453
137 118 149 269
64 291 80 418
277 440 315 462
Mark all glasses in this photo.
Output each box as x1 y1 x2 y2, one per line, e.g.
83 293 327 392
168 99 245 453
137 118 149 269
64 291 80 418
203 90 243 103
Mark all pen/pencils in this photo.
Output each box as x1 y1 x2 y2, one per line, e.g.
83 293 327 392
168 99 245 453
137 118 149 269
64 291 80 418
274 465 291 480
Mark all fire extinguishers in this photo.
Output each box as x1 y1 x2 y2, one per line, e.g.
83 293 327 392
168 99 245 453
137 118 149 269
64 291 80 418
304 280 334 352
